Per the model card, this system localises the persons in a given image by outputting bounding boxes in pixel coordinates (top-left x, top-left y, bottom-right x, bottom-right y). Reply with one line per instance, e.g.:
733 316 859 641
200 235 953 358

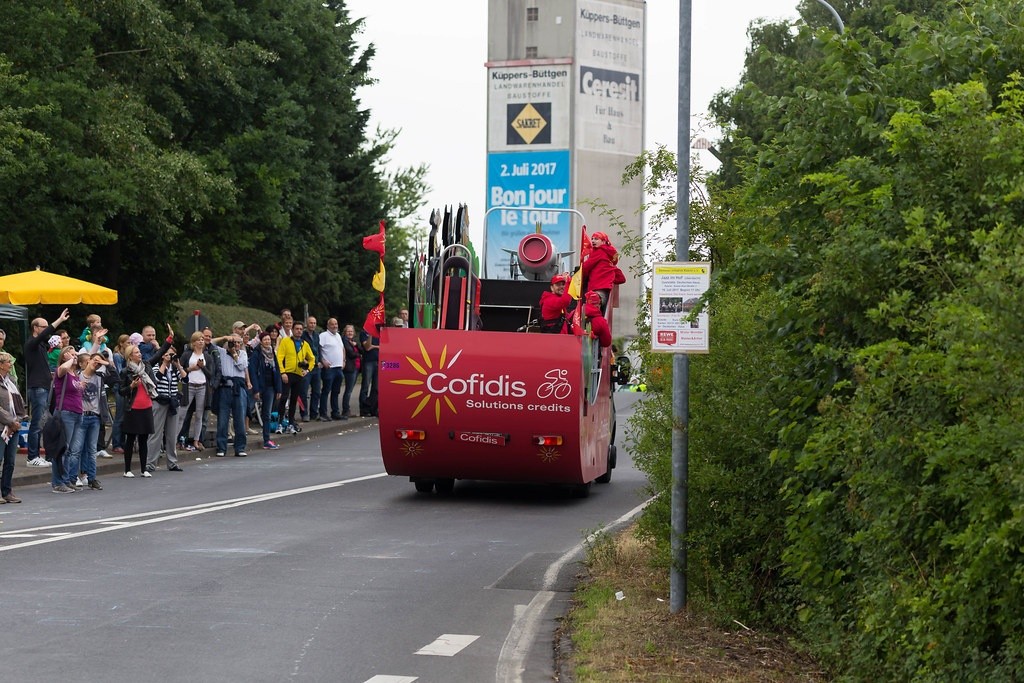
557 290 612 347
538 274 572 334
582 232 626 315
0 307 410 504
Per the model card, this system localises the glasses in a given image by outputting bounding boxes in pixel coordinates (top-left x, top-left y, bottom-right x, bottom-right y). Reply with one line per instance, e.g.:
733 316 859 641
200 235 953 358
38 326 47 329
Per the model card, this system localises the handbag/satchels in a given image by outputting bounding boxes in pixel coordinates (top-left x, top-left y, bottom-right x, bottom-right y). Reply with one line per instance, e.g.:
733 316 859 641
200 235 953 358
540 318 563 334
42 414 67 462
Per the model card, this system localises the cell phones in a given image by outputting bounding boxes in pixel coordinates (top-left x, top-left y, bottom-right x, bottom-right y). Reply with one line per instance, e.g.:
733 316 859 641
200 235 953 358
133 374 139 381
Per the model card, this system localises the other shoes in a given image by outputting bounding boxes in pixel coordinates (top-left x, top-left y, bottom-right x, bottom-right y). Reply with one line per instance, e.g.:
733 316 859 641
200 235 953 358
239 452 247 456
99 450 113 458
81 476 89 486
75 477 83 487
216 453 224 456
169 466 183 471
114 413 379 454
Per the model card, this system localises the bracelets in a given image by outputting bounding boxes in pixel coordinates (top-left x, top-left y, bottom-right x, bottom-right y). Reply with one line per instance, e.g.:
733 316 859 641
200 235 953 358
233 354 237 358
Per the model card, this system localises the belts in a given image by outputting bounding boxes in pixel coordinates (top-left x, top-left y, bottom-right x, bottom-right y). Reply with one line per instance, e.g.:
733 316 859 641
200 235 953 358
190 383 205 387
82 411 100 417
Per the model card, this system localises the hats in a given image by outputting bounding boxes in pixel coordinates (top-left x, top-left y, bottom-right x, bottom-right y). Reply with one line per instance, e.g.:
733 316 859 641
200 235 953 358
551 275 566 284
232 321 247 331
585 290 601 305
260 331 269 341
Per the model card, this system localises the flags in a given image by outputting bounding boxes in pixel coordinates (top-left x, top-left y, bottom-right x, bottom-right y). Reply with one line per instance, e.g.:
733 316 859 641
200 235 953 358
361 221 385 338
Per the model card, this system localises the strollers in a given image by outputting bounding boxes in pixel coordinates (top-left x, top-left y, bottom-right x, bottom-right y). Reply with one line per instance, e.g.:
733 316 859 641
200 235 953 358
250 395 302 433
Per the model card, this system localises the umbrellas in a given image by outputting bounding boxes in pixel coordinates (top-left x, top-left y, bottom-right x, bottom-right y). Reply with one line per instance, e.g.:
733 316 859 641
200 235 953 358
0 265 118 318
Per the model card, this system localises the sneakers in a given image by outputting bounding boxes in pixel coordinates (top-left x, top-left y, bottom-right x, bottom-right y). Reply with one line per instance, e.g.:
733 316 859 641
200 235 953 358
38 457 52 466
89 480 103 490
0 498 6 504
141 471 152 477
66 483 83 492
26 457 49 467
52 485 76 493
123 471 134 477
3 494 22 503
263 439 279 451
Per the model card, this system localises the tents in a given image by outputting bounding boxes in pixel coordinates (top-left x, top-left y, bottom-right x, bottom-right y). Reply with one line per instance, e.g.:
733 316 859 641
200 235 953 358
0 304 28 418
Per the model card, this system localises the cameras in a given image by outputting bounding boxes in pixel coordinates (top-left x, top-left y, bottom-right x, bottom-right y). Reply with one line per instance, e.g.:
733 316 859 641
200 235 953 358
228 341 234 349
169 353 181 363
298 362 309 370
